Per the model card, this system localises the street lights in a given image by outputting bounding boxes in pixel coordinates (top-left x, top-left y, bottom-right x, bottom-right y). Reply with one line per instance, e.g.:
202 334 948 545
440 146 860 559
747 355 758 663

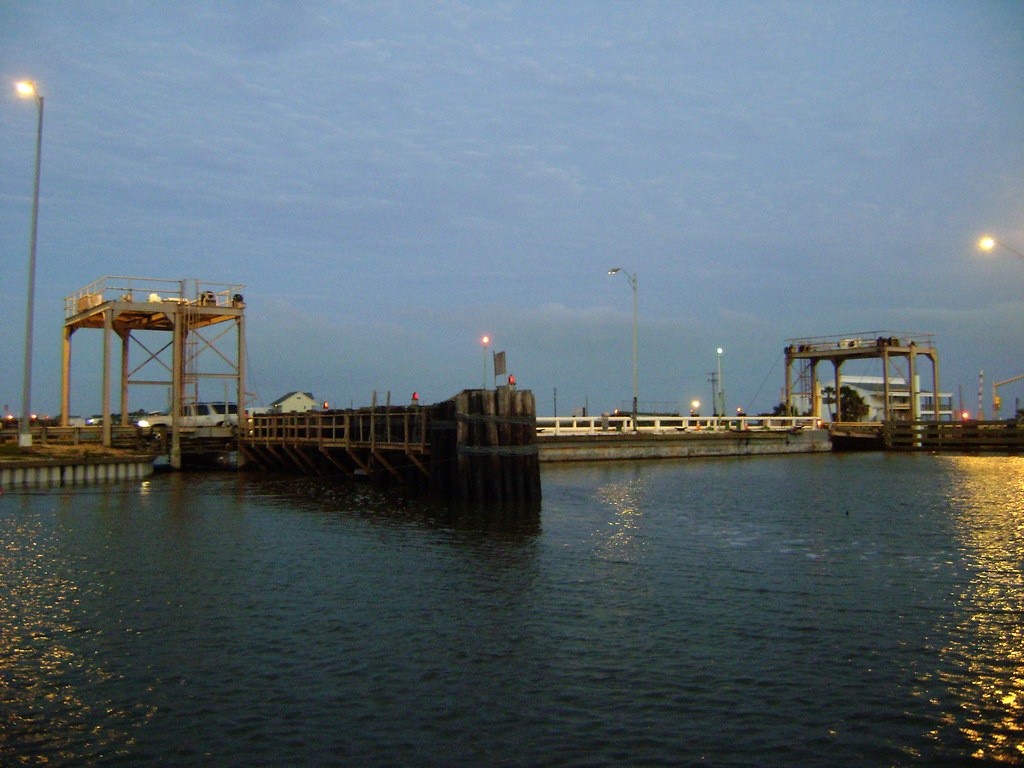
482 336 489 388
717 348 724 417
12 82 45 447
608 267 638 430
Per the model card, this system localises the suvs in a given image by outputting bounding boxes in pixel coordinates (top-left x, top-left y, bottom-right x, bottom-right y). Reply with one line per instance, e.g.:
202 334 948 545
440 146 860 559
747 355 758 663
135 400 247 437
